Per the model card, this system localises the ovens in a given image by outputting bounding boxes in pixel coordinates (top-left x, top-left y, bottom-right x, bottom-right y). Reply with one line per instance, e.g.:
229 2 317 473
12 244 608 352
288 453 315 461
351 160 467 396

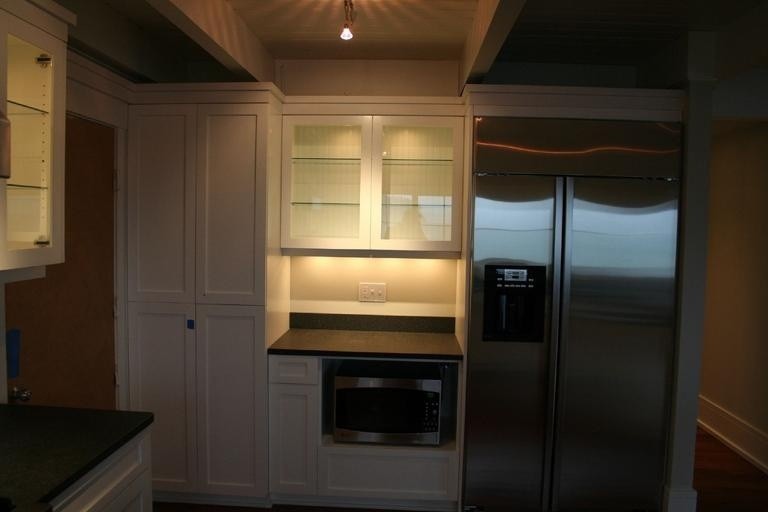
332 360 444 446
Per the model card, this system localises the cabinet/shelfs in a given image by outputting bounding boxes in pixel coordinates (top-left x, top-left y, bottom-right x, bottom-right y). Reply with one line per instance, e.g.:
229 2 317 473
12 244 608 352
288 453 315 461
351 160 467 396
122 83 293 509
0 0 79 273
268 351 464 512
280 96 466 254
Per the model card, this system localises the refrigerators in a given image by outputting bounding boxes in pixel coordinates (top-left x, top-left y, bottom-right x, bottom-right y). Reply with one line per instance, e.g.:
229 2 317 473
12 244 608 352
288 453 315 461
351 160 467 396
465 111 684 511
